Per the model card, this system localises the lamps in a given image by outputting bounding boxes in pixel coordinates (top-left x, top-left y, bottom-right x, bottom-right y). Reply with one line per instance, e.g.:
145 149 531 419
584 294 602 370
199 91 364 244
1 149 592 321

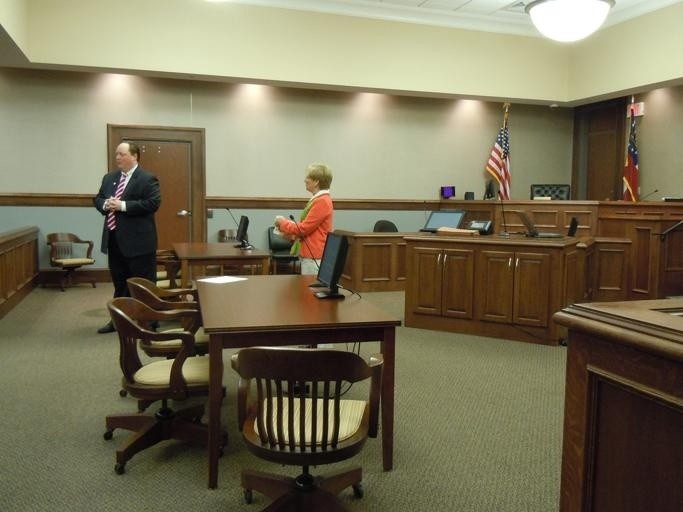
523 0 617 44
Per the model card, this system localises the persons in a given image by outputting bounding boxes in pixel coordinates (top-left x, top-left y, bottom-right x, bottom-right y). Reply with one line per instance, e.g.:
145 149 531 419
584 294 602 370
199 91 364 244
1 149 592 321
93 139 160 334
273 165 334 274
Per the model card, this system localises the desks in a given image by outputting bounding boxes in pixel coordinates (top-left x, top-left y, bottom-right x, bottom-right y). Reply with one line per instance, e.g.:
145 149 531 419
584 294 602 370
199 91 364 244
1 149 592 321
553 297 683 512
195 274 403 489
168 243 272 286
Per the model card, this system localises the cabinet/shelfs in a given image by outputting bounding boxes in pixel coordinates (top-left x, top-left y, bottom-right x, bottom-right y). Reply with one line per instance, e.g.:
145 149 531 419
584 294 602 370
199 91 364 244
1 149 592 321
334 229 409 295
403 235 577 346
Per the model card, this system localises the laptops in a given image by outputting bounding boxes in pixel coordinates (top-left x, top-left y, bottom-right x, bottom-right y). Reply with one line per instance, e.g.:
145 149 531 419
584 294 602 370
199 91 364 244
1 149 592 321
515 210 563 238
420 211 466 232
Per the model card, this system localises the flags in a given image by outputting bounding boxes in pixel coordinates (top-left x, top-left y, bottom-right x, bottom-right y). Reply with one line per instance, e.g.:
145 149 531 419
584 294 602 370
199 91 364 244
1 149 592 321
486 110 511 201
622 103 640 202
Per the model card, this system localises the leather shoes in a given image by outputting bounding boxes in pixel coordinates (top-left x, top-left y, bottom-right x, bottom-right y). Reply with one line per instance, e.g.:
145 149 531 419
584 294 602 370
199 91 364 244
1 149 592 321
98 318 116 334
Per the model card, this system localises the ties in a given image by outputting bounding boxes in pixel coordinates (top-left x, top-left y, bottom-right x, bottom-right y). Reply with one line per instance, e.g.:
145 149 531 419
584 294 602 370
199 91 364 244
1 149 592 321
106 173 128 232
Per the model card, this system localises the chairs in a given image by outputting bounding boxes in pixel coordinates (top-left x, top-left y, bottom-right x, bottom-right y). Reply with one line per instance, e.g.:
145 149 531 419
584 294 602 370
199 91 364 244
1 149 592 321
38 232 96 292
119 278 226 403
105 297 228 476
568 217 578 236
266 228 301 273
224 349 393 511
529 184 570 200
151 249 182 290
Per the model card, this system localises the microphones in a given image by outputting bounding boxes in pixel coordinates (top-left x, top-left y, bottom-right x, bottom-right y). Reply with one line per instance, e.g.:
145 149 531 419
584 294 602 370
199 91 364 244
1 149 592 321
226 207 254 250
497 190 508 236
637 189 658 202
288 215 327 288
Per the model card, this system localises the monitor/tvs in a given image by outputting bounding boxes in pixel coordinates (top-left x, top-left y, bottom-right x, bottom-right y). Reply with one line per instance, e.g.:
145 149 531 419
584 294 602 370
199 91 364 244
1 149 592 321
233 216 249 248
314 232 350 299
